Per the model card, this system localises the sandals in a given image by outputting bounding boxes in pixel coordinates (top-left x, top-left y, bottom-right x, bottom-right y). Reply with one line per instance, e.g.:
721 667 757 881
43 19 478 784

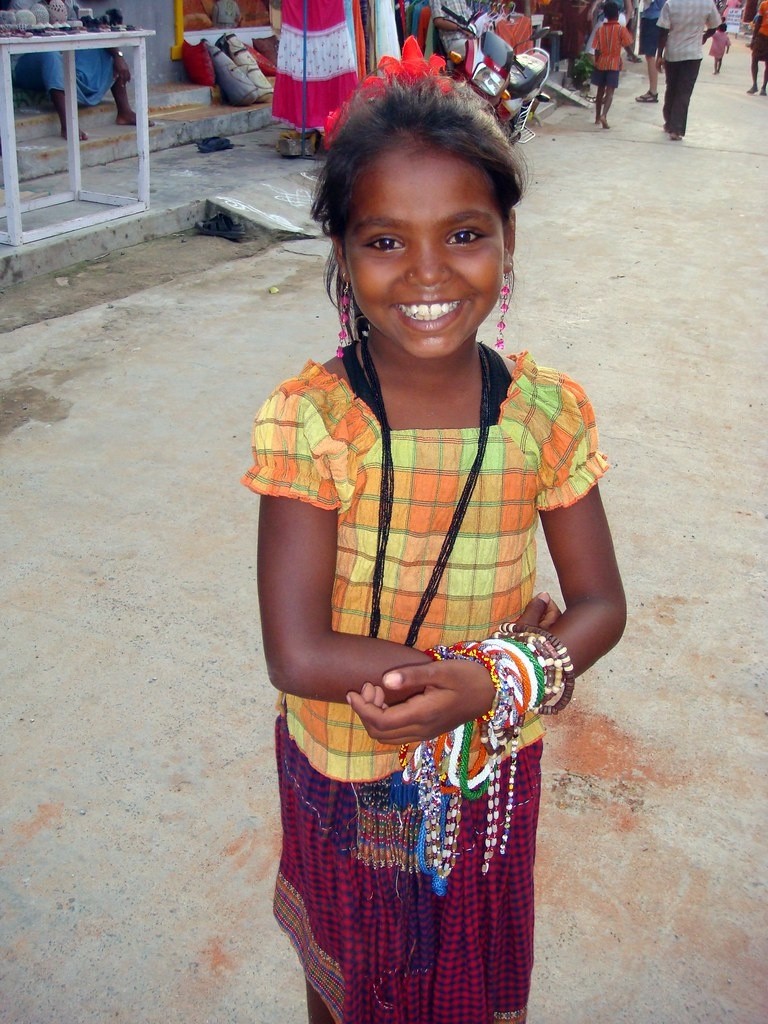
635 90 659 102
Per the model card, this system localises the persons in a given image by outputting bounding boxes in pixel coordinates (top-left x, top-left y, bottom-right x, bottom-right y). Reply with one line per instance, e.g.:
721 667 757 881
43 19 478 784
591 3 638 129
709 23 731 74
8 0 155 141
747 0 768 96
635 0 667 103
242 35 628 1024
655 0 722 140
608 0 641 62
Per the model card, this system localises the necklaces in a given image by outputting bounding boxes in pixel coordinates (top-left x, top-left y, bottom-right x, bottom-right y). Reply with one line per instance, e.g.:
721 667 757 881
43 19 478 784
398 623 575 897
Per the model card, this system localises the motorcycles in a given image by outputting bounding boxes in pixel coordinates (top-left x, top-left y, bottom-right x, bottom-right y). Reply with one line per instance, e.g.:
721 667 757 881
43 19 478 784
439 5 550 147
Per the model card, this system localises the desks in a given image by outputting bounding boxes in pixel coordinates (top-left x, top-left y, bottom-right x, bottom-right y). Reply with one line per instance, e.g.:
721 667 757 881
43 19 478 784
0 29 156 244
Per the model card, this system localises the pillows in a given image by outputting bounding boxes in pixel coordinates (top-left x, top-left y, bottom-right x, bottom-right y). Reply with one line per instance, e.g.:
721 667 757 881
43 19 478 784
243 42 276 77
208 33 273 107
251 35 277 66
182 39 215 87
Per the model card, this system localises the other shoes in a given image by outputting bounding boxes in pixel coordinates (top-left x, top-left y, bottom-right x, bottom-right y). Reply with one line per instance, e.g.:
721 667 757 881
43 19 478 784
627 56 642 63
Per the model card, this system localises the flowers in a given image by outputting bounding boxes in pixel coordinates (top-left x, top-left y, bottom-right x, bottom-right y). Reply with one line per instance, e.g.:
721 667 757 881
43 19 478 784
324 35 451 151
